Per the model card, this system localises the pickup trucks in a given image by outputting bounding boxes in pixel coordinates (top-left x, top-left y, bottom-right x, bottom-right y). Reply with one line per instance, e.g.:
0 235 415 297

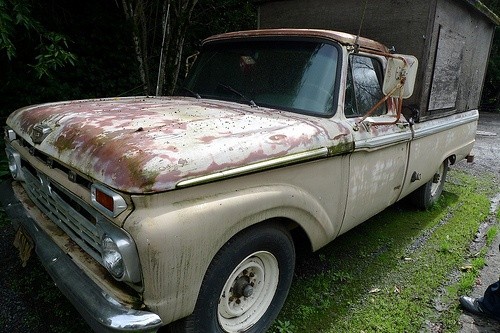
9 27 479 333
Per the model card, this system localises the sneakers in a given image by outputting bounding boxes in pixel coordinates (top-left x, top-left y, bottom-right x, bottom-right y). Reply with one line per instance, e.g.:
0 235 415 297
459 296 488 317
